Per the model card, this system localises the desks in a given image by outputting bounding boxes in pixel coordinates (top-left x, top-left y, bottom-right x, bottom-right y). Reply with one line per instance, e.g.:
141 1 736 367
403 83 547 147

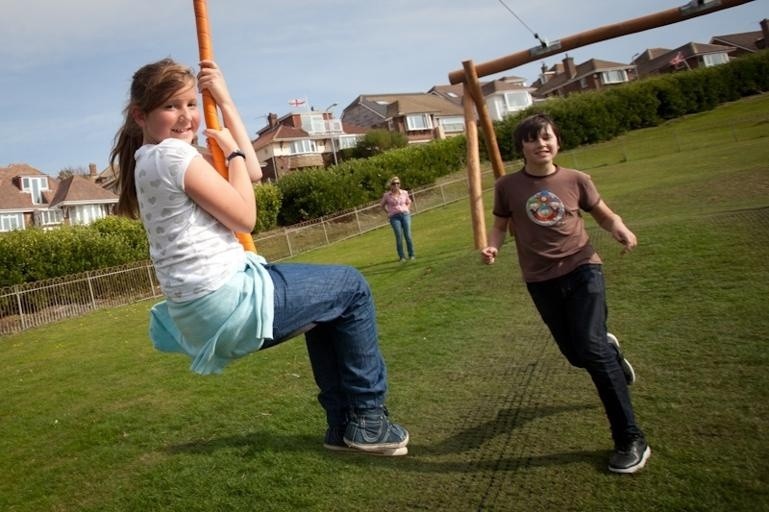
325 102 339 167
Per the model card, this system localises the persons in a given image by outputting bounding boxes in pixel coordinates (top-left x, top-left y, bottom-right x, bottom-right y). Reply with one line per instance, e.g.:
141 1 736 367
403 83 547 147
108 57 412 456
381 176 414 263
481 111 652 473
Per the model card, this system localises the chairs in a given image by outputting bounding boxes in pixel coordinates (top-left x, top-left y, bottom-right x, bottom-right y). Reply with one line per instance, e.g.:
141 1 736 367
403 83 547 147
601 331 635 386
607 435 652 474
323 420 411 456
338 406 411 449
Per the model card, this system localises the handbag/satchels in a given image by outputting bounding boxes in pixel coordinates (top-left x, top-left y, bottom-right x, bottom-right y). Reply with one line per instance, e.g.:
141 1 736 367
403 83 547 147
224 148 247 166
